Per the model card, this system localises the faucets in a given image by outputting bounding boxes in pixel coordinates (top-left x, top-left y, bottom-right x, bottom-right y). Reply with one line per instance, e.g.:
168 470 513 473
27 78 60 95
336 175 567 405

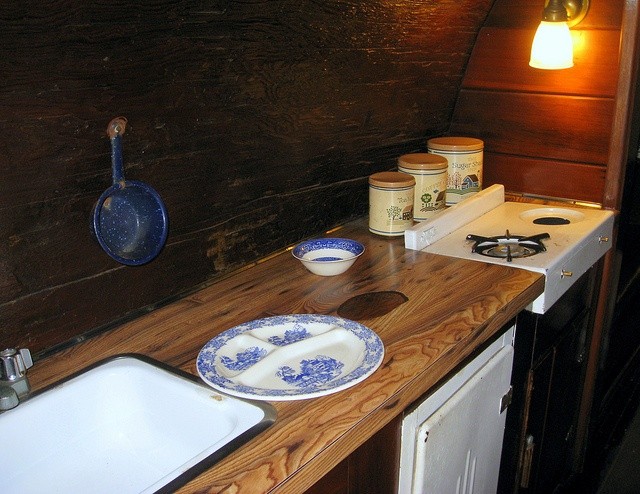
0 346 33 411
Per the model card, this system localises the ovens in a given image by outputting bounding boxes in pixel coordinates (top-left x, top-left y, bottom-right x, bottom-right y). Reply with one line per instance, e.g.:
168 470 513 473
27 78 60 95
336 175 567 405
497 254 606 494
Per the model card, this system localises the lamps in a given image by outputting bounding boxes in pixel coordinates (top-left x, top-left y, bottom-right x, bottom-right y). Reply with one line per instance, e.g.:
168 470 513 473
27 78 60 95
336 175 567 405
527 0 591 74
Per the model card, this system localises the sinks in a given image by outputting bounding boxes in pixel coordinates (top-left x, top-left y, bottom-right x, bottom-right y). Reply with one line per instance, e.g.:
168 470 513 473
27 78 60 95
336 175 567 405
0 357 265 494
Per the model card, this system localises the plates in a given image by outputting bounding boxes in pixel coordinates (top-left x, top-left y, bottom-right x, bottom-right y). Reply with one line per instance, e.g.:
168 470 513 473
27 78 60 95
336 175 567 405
196 312 385 401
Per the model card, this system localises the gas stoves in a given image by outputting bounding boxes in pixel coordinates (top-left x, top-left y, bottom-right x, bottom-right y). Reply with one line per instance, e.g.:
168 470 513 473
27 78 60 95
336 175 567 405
421 201 616 315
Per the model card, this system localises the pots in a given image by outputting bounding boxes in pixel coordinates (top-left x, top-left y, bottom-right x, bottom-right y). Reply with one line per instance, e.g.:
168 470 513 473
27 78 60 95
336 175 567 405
87 117 170 267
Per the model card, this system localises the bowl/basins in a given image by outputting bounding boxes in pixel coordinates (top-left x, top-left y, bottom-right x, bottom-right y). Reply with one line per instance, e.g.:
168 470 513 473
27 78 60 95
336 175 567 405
291 238 366 277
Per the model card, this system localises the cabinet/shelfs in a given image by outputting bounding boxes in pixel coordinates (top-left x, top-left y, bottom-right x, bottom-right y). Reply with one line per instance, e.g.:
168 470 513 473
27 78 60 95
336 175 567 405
303 318 544 492
513 257 607 492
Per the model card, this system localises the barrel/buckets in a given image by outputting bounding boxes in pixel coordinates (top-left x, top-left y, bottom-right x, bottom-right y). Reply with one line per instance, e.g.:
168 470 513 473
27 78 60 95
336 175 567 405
427 136 486 206
397 152 447 221
367 169 415 238
